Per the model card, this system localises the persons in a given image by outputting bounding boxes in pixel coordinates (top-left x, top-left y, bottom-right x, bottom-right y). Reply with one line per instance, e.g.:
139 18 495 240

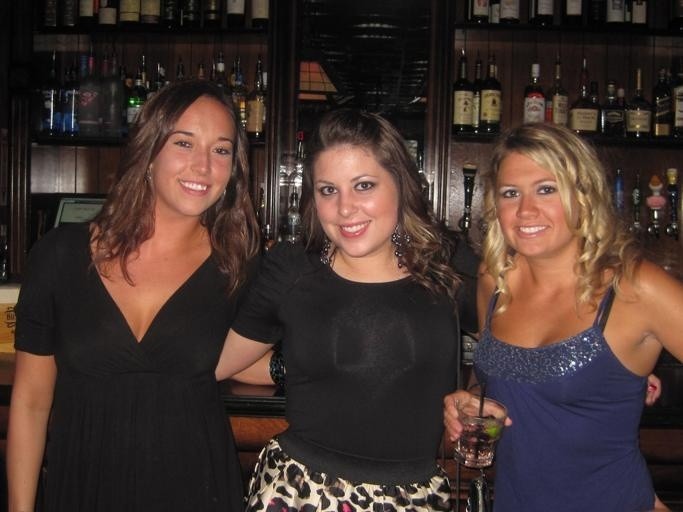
213 97 664 512
442 122 682 511
3 79 290 509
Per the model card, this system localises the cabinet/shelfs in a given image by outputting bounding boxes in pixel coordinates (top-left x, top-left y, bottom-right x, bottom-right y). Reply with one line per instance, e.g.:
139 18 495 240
0 1 683 335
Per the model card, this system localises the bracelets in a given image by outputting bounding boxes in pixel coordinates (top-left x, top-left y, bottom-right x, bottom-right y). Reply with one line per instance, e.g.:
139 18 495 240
267 351 285 390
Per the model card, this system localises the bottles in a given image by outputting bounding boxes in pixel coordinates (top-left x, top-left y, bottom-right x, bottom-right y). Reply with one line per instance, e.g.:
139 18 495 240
39 0 270 143
452 46 683 139
282 126 314 247
464 0 652 32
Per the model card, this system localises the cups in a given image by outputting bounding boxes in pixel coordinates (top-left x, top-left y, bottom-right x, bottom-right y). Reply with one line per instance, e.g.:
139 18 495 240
452 394 508 470
